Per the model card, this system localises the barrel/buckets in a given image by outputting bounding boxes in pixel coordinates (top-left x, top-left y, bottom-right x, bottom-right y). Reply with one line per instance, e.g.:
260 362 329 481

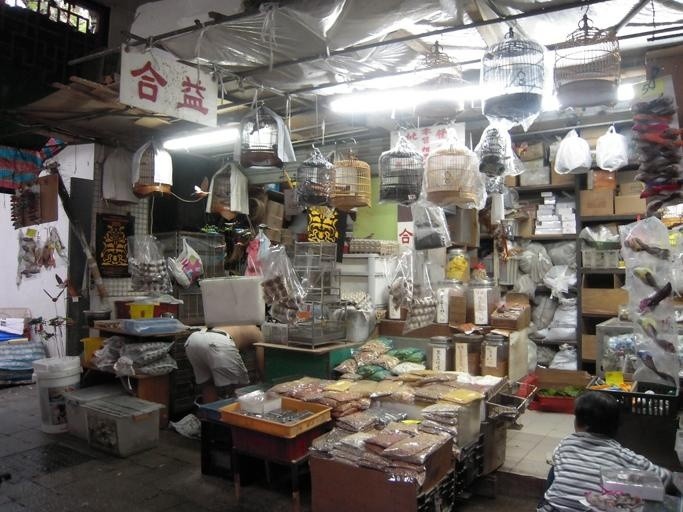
32 356 82 434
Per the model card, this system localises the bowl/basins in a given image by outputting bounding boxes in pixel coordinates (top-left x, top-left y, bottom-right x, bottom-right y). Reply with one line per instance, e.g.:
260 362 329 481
83 309 111 328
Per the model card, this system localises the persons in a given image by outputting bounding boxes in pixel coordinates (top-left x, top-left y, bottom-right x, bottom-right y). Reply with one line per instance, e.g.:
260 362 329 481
183 324 264 404
534 389 672 512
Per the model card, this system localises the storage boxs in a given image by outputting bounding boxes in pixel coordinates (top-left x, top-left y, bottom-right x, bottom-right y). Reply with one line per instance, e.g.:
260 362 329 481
486 125 650 390
63 380 122 442
532 387 577 411
483 415 510 476
414 435 462 496
84 395 165 457
151 185 448 417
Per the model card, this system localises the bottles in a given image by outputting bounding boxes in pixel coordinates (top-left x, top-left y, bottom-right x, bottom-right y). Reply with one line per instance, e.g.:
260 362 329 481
436 281 500 326
429 333 508 378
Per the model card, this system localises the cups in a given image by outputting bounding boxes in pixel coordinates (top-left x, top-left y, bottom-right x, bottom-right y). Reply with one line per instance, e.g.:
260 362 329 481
80 337 101 364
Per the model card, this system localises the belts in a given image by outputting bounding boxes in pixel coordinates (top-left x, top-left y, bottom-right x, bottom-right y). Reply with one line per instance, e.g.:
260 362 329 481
190 328 233 340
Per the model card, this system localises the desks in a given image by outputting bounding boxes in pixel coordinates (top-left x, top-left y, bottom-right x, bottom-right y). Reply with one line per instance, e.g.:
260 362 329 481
224 429 334 510
80 366 169 427
